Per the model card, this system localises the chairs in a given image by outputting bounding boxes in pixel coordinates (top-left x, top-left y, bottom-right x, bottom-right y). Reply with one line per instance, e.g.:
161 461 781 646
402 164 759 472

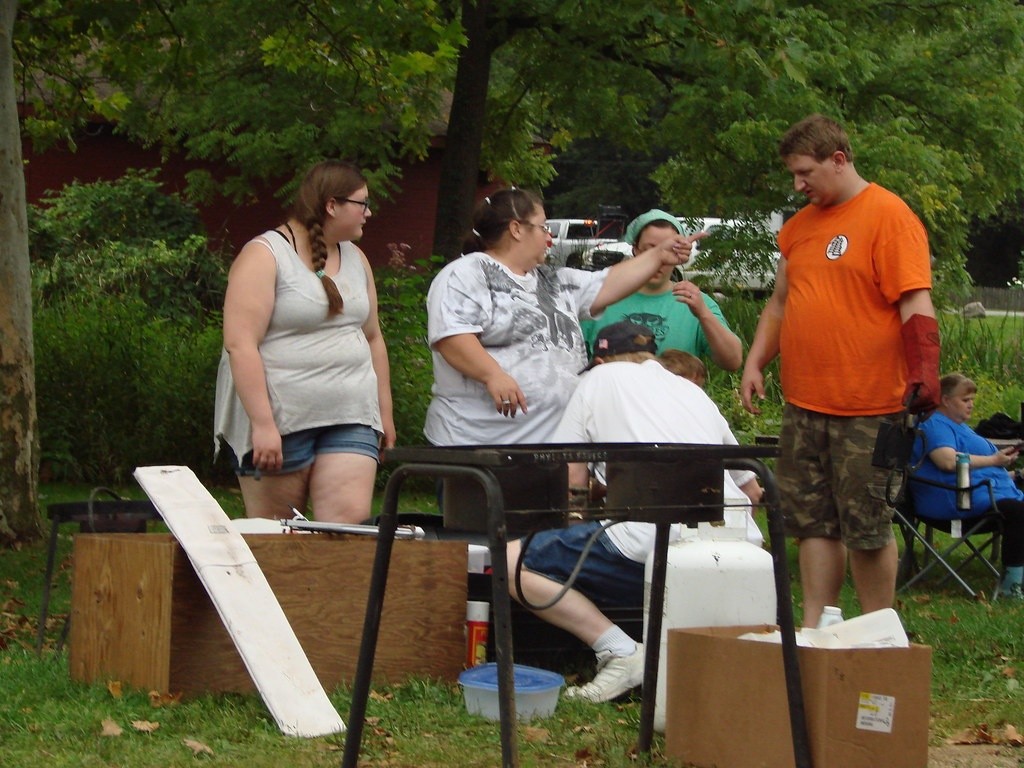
888 470 1002 599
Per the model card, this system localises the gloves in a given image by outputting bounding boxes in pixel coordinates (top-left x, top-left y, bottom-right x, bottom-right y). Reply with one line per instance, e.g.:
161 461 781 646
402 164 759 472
901 314 941 422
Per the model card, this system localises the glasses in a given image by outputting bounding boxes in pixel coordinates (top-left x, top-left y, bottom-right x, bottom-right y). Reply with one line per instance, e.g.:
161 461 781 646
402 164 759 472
518 219 551 234
339 198 371 214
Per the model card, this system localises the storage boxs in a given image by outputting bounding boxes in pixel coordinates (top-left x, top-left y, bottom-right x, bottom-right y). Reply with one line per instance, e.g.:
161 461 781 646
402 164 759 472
69 531 469 704
664 622 932 768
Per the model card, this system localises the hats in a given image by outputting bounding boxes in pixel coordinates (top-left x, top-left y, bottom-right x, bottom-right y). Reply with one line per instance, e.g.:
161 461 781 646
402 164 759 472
576 322 658 375
625 209 687 244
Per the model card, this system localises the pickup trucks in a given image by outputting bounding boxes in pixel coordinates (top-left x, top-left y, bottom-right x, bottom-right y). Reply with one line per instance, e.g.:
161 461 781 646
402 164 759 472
581 217 781 301
544 217 619 270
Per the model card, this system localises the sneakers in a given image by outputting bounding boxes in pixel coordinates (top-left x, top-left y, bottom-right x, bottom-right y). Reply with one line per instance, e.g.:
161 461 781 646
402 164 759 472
563 643 644 704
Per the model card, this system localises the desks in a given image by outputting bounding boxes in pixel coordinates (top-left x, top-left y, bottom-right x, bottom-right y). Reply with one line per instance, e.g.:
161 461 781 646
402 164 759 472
922 438 1024 566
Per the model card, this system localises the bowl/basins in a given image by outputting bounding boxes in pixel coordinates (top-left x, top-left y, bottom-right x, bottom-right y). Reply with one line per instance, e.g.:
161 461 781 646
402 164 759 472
457 664 566 719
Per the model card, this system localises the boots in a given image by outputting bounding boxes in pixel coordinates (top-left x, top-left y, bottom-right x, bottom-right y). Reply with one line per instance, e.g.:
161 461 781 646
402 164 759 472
1000 566 1024 599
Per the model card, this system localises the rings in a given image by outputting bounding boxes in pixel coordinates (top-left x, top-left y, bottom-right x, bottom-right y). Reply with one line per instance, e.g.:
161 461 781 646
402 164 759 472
504 400 511 404
690 294 692 298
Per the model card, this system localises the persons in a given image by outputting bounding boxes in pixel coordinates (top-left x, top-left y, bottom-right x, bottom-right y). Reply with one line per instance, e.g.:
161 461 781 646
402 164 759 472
506 322 766 704
906 371 1024 596
579 209 743 372
423 187 709 516
211 162 398 524
740 112 942 629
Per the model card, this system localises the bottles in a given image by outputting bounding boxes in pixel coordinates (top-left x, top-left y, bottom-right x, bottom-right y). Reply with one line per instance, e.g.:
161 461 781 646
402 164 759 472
956 454 970 509
